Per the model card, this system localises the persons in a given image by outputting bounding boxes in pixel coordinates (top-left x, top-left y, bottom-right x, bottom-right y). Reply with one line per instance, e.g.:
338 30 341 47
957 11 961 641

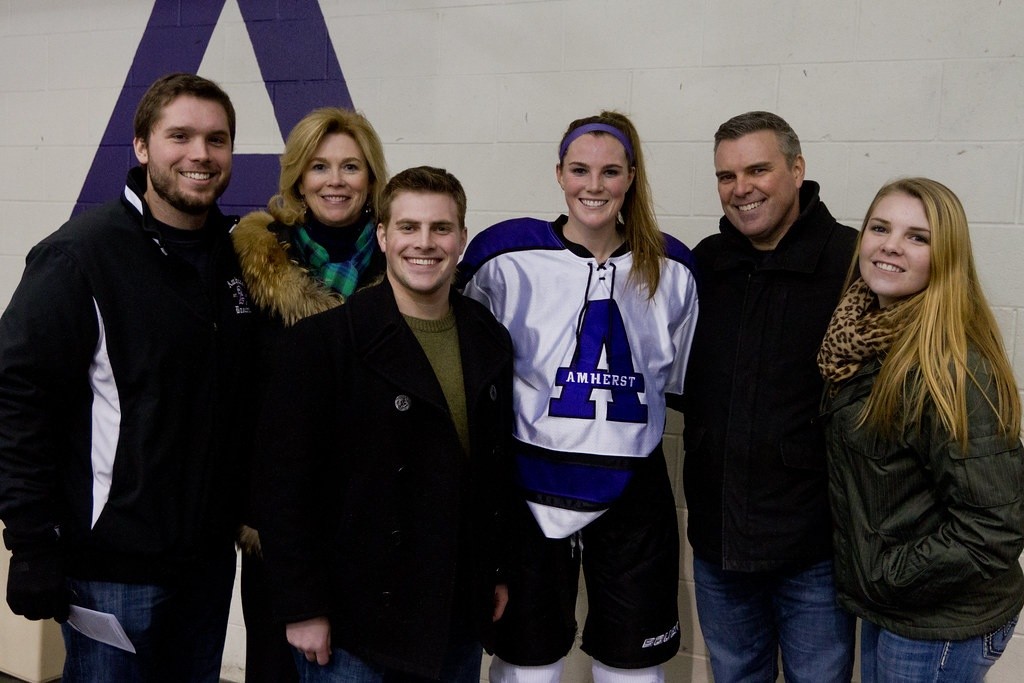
0 72 239 683
455 110 701 682
811 174 1024 682
224 106 512 683
685 110 861 666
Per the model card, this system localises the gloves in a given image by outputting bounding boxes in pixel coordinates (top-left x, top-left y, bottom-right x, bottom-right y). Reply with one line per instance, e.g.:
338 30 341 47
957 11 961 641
1 526 81 625
